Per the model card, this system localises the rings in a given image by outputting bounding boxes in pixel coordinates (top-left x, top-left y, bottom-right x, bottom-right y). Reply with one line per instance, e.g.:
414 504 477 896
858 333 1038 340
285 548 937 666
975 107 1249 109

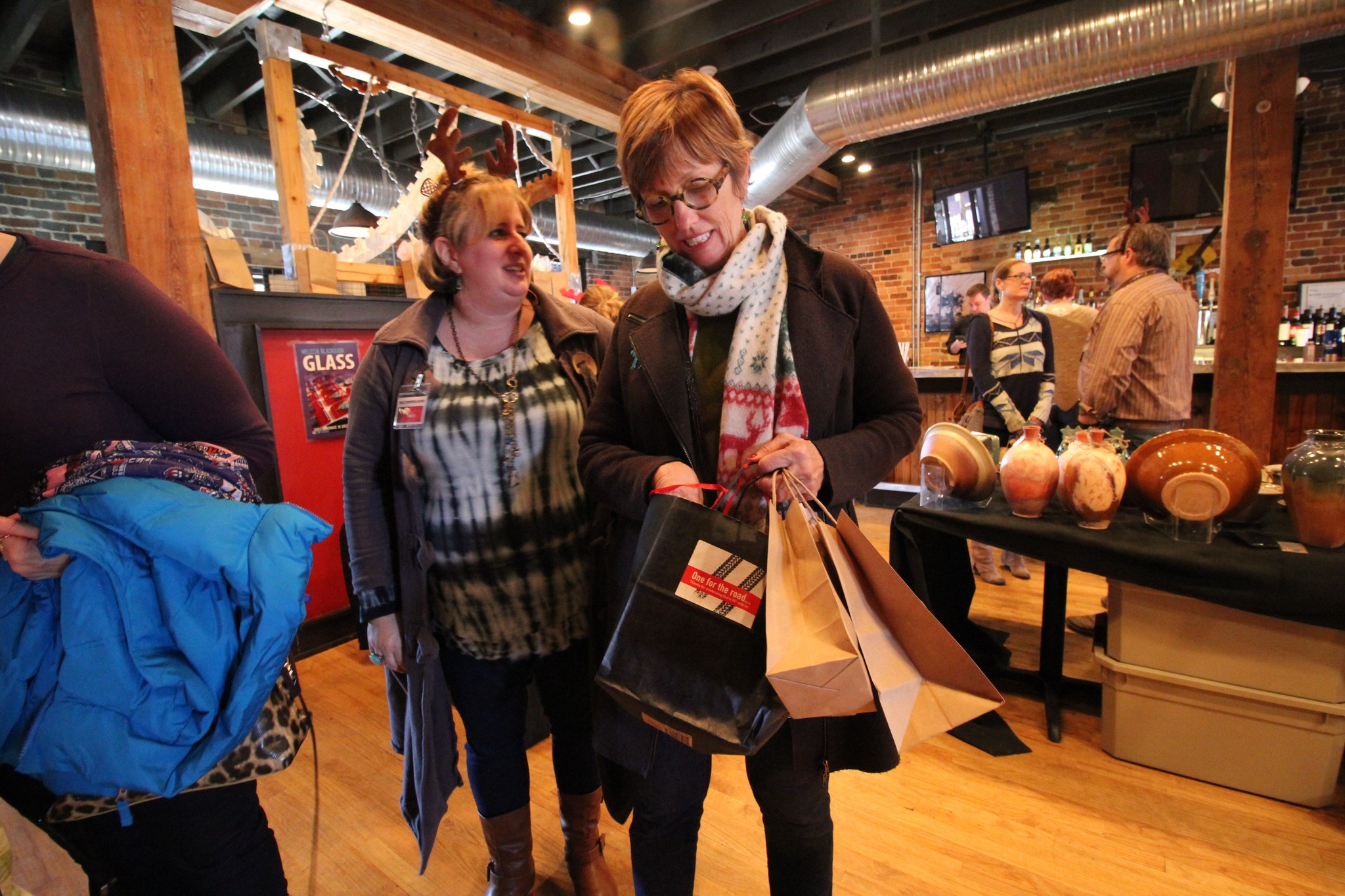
368 653 385 665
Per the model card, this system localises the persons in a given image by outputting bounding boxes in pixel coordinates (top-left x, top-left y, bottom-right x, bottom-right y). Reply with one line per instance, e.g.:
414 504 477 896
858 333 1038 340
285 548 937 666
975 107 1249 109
568 67 922 894
944 284 995 366
580 284 623 325
339 162 622 896
1030 268 1098 430
1067 222 1199 640
0 226 289 896
966 257 1060 584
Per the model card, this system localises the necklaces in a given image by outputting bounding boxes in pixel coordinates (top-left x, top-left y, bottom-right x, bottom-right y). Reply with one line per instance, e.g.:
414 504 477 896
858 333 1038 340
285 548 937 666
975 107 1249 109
446 297 525 489
989 312 1022 372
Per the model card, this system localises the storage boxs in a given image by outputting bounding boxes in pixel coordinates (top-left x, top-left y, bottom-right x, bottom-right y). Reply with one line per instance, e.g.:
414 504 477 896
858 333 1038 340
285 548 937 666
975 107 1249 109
1100 577 1345 705
1091 640 1345 810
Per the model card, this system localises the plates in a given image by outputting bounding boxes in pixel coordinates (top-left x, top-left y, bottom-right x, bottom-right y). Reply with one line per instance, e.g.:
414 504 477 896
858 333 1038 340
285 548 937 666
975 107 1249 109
1258 467 1284 494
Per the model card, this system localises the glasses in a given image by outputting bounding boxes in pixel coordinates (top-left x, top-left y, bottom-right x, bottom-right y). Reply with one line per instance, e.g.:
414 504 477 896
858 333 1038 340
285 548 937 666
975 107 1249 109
1002 272 1033 280
1099 248 1125 261
635 161 731 226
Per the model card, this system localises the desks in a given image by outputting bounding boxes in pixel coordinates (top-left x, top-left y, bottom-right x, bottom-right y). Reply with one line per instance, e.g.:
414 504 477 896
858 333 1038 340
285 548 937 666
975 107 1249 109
895 481 1345 745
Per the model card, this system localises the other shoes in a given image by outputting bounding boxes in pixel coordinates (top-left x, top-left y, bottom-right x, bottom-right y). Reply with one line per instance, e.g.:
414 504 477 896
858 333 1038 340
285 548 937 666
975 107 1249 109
1064 610 1108 635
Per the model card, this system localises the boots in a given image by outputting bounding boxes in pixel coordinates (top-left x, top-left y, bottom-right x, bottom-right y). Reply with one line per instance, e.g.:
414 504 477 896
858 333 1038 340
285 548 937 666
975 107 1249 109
476 797 536 896
970 539 1006 586
555 783 620 896
1001 549 1030 580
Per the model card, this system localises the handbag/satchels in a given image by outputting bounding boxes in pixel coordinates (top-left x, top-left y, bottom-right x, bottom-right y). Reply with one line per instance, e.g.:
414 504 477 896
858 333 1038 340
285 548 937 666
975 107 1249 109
41 645 313 824
951 399 984 434
783 467 1005 756
595 460 789 755
767 470 878 721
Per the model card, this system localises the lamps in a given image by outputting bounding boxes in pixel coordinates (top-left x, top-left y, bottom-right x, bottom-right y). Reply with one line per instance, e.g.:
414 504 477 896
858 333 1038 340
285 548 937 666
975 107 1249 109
1295 77 1311 99
1210 92 1230 113
567 0 598 27
327 76 381 241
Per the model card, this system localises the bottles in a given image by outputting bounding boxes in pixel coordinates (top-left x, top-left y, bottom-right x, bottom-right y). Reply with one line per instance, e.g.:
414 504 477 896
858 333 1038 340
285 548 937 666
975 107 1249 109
1278 302 1345 358
1023 287 1044 308
1014 233 1092 260
1282 429 1345 548
1000 425 1127 530
1077 286 1105 310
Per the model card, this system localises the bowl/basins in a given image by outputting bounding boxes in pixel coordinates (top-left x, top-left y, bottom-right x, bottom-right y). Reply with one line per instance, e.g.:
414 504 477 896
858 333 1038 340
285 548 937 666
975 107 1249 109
918 422 997 499
1125 429 1261 523
